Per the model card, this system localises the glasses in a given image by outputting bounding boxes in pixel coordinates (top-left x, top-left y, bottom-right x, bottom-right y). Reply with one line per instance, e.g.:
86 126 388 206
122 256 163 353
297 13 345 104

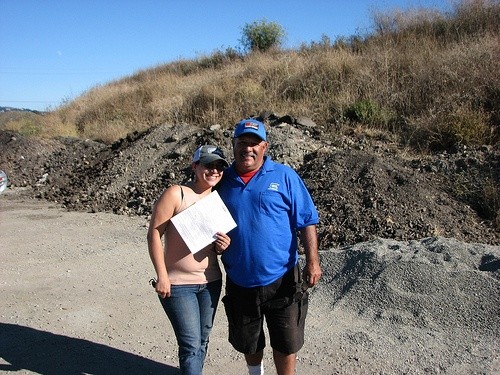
201 163 225 172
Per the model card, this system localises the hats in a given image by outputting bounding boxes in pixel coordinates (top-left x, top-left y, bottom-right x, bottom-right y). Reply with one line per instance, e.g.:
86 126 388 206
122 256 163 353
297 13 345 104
193 145 230 168
234 119 267 143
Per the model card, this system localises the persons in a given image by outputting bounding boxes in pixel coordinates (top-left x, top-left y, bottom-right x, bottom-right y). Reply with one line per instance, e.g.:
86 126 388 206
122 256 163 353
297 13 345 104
212 118 322 375
147 144 232 375
